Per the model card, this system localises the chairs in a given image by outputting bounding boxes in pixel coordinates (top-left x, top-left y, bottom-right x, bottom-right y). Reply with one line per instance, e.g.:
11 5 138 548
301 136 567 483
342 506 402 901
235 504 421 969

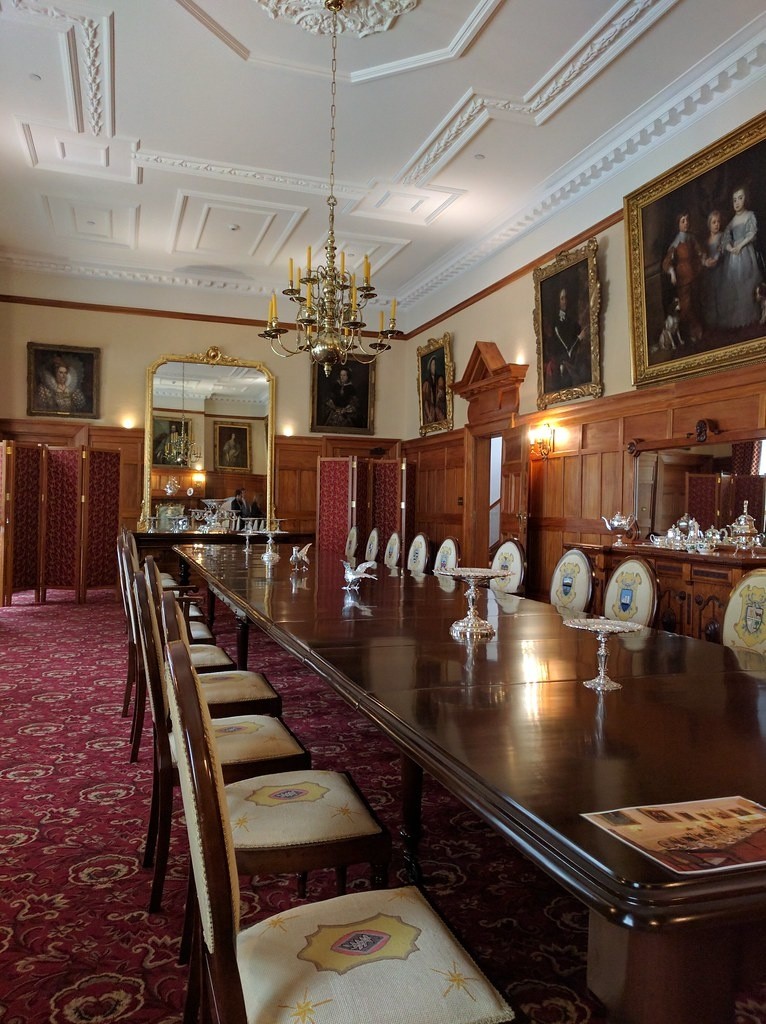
111 530 766 1023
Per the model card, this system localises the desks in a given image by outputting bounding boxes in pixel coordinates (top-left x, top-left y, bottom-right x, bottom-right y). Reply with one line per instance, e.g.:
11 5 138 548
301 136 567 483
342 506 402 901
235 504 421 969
133 532 316 561
173 543 766 1024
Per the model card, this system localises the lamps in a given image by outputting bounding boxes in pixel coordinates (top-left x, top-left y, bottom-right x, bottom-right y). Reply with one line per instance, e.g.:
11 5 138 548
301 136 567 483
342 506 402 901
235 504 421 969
528 424 552 461
256 1 405 378
163 361 202 468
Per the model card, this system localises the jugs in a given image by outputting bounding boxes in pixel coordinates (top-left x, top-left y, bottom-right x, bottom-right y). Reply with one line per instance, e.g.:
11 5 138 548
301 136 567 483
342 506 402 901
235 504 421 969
602 511 636 546
700 525 728 543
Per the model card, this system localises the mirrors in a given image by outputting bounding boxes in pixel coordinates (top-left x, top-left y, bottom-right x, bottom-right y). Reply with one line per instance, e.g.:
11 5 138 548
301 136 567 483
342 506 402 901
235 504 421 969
136 346 279 533
628 418 766 555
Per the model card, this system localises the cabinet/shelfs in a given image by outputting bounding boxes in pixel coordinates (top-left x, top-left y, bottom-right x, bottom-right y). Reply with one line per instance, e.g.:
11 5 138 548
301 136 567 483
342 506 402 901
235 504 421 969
563 541 766 646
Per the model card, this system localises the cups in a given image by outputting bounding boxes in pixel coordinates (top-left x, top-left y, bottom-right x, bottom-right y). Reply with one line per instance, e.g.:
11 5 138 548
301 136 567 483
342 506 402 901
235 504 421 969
697 543 716 555
650 534 666 546
686 543 697 554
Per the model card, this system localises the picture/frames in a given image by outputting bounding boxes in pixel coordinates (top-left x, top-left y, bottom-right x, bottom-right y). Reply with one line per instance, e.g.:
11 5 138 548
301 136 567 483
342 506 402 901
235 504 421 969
416 332 455 436
309 353 376 435
152 415 192 467
213 421 253 474
532 237 603 410
622 109 766 388
26 341 100 419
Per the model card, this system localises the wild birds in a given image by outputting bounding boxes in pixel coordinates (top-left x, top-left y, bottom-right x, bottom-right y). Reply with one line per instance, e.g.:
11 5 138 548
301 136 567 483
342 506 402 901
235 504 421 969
290 543 313 572
339 560 378 590
